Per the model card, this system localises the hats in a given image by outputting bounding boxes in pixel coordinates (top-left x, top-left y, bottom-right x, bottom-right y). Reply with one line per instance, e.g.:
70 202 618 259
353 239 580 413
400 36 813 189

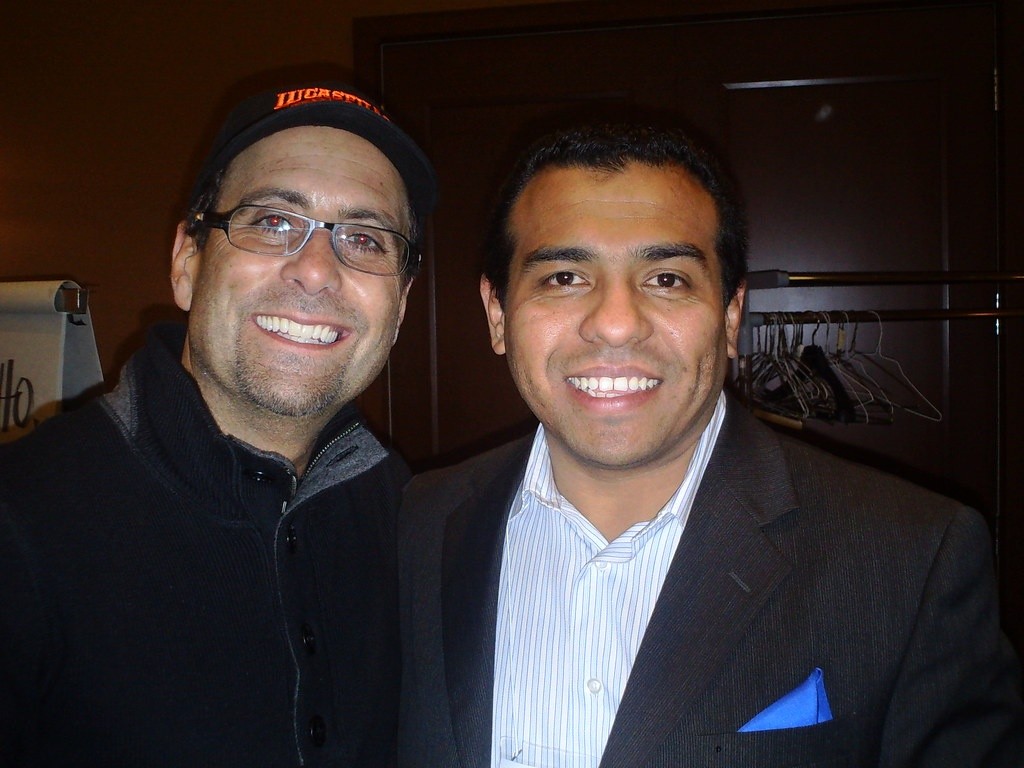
188 84 435 215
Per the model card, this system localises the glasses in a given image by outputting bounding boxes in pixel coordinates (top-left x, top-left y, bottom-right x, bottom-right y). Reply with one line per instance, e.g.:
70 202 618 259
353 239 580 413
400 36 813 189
193 203 421 277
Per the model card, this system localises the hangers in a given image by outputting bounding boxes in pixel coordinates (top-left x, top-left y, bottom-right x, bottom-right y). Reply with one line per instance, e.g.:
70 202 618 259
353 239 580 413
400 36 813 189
725 311 943 430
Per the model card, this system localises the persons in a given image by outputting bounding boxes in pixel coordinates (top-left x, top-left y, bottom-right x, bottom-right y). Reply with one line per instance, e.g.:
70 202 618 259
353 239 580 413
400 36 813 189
393 120 1024 768
0 59 433 768
